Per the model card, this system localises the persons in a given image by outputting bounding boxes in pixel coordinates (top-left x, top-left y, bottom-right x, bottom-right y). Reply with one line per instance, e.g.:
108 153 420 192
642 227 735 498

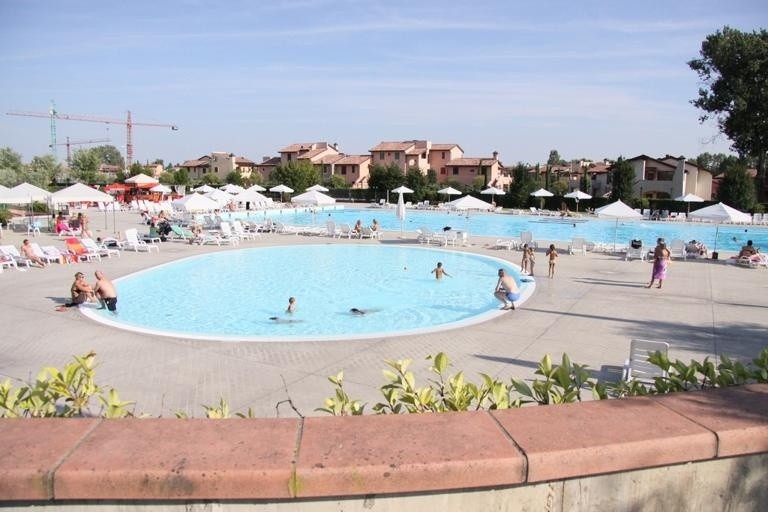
521 244 535 276
494 269 520 310
740 240 756 253
649 238 670 288
77 212 85 231
546 244 558 278
70 271 117 311
21 239 47 269
355 220 361 232
50 211 70 232
288 297 299 314
372 219 378 231
431 262 451 280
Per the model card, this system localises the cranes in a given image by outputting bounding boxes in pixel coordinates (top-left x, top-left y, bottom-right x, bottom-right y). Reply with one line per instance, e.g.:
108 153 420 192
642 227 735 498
5 98 179 175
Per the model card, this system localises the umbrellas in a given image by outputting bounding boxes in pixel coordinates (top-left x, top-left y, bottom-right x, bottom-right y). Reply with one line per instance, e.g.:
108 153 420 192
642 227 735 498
103 173 336 239
0 180 114 225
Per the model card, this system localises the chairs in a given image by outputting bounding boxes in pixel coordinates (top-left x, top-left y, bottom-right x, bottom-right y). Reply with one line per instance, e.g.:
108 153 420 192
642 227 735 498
492 230 537 252
669 238 688 260
264 214 386 240
624 239 645 261
621 338 670 396
116 228 160 254
1 214 122 269
145 214 264 247
415 227 471 249
567 236 588 257
